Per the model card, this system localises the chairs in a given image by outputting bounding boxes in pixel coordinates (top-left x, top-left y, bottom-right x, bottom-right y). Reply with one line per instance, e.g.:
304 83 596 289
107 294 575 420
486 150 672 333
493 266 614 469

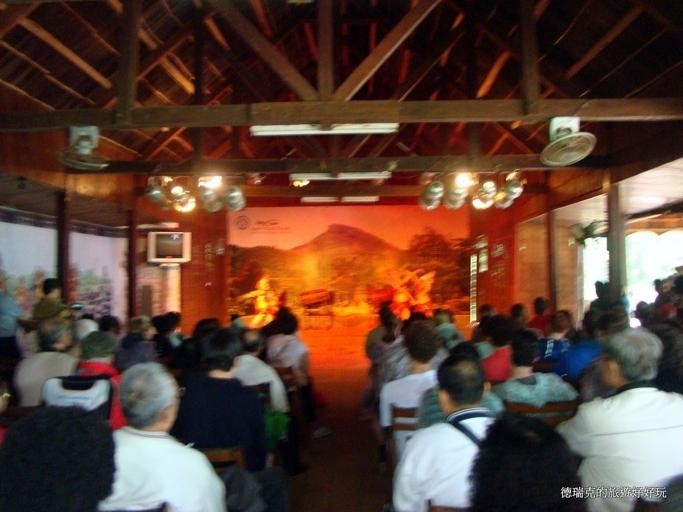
506 400 579 431
204 446 245 479
276 364 303 413
386 406 419 485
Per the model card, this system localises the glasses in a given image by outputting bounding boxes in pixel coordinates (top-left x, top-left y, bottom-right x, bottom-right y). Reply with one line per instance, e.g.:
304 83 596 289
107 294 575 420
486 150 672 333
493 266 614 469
175 387 186 397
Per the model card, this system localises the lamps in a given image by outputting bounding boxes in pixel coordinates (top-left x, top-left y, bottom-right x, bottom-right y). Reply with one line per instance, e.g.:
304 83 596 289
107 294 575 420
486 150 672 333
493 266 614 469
249 122 399 135
471 180 497 210
420 181 444 210
445 171 480 208
224 186 246 211
495 169 524 210
289 170 392 180
198 176 224 212
146 176 196 212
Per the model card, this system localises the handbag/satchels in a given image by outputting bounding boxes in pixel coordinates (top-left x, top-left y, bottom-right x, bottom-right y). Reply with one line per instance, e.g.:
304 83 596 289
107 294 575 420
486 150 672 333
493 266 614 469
447 420 482 447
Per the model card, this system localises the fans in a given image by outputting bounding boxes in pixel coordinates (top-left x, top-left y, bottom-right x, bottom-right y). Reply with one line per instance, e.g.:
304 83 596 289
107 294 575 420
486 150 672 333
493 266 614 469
58 126 109 169
540 116 596 167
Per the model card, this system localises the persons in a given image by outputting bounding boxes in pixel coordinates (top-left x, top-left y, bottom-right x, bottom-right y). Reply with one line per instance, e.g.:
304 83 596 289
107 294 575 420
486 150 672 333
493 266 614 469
261 308 288 336
14 317 79 407
499 333 579 415
0 403 115 511
537 310 573 361
473 323 495 358
98 360 228 511
68 318 99 356
71 331 126 429
416 340 506 425
526 296 553 336
169 332 290 510
194 319 220 336
118 316 161 370
365 303 464 382
264 317 318 422
466 409 584 511
231 329 306 475
556 320 599 381
471 304 495 340
30 279 70 322
82 315 121 358
582 266 683 387
0 372 13 445
553 329 682 512
391 352 497 512
159 312 186 365
478 316 513 383
508 303 526 324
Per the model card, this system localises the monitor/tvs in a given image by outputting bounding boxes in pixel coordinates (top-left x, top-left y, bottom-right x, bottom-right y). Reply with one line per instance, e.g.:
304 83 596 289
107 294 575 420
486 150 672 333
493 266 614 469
146 231 191 266
